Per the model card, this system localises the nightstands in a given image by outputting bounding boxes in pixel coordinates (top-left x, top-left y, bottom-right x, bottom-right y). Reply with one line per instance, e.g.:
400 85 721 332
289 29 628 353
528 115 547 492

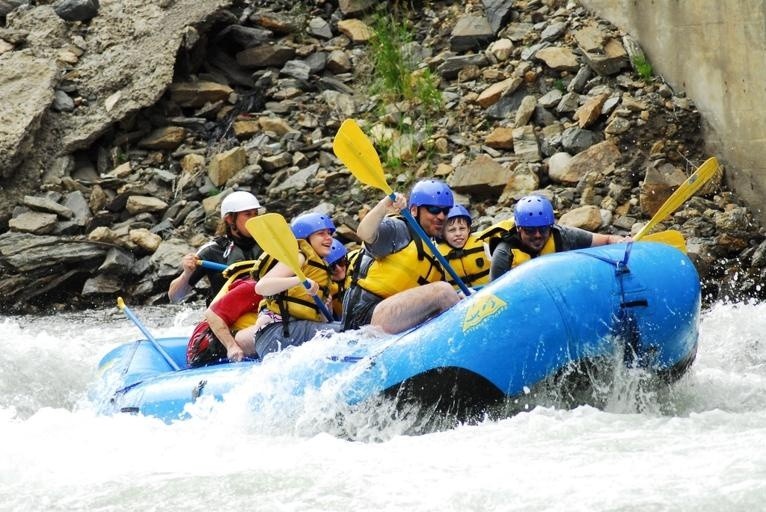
333 118 471 298
246 213 335 320
633 158 719 240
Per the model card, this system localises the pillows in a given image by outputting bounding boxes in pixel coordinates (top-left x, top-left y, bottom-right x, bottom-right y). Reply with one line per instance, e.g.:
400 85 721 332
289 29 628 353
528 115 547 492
220 191 261 218
514 195 555 226
408 177 453 210
448 205 472 223
323 238 348 266
291 212 336 240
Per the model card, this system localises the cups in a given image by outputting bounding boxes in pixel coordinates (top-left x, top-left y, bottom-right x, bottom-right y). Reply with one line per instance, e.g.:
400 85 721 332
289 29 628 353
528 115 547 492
331 258 345 267
524 227 549 235
425 206 449 215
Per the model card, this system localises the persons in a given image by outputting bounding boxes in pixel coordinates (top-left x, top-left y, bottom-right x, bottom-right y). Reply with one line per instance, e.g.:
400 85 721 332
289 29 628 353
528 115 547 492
490 195 633 283
186 275 263 364
322 238 361 321
254 212 341 360
169 191 263 309
434 203 492 291
340 179 478 336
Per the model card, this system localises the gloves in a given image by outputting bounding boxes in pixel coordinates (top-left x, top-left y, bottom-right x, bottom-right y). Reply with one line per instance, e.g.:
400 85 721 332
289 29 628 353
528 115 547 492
90 238 702 437
